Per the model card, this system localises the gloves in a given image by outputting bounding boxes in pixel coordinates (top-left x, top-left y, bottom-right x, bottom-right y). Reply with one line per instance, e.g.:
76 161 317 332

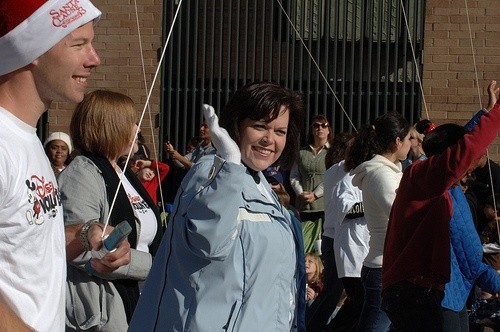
200 103 241 165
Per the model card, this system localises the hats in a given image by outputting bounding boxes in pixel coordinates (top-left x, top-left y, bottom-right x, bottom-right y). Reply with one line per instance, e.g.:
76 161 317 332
43 131 73 156
0 0 103 80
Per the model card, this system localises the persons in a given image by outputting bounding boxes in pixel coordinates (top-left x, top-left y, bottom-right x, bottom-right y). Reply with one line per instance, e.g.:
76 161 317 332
43 131 72 177
127 82 307 332
118 125 294 236
302 252 327 309
0 0 101 332
55 89 163 331
349 111 412 331
331 135 425 331
304 134 357 332
382 80 500 332
165 118 216 168
290 114 342 255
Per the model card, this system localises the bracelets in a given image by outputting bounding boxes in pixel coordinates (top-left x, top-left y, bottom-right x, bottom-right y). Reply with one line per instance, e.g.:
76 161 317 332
79 219 96 253
313 192 319 200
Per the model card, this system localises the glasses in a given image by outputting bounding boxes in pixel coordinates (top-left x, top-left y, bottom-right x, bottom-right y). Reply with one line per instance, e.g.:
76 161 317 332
311 122 329 129
